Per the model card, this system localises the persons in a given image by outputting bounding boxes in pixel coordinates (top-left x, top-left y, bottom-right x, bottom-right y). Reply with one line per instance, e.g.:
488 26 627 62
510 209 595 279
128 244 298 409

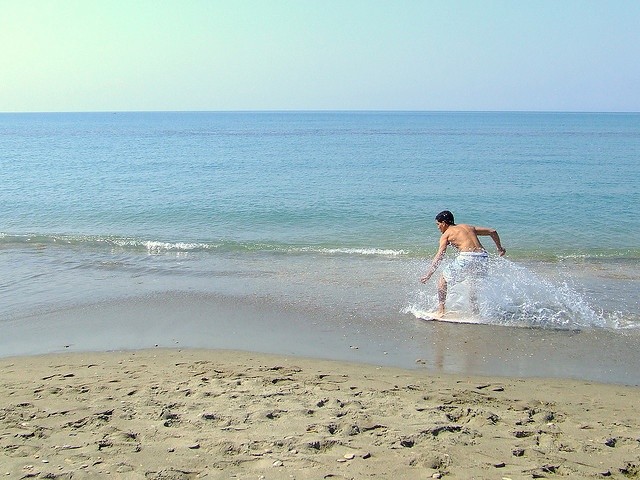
421 210 506 316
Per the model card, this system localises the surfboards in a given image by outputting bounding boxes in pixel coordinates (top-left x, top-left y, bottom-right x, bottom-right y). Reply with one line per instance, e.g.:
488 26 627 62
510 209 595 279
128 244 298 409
412 309 583 331
